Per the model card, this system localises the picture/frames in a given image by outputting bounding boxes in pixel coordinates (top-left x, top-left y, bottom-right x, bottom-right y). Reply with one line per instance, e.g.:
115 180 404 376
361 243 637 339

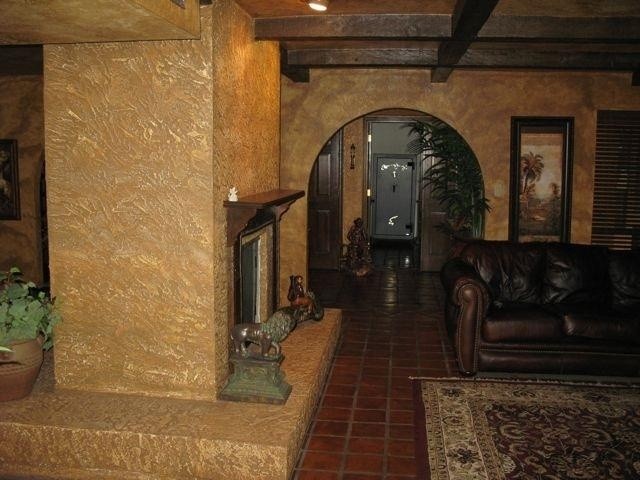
0 139 22 221
507 115 575 244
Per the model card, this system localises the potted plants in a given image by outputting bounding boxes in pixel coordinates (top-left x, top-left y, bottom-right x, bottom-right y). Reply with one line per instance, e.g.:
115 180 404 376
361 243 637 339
0 262 64 403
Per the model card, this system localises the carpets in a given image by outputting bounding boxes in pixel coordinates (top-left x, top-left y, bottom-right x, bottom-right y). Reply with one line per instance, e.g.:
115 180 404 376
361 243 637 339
407 374 640 480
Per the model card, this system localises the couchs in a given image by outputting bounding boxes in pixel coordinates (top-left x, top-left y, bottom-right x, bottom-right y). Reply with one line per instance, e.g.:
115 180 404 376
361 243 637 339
439 237 640 379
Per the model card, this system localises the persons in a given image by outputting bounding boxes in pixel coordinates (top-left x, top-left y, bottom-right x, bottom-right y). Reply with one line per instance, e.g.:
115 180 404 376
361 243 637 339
347 218 368 259
290 274 313 314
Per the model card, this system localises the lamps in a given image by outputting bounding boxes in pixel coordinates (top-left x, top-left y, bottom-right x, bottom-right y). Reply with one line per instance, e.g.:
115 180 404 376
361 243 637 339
350 144 356 169
308 0 330 12
